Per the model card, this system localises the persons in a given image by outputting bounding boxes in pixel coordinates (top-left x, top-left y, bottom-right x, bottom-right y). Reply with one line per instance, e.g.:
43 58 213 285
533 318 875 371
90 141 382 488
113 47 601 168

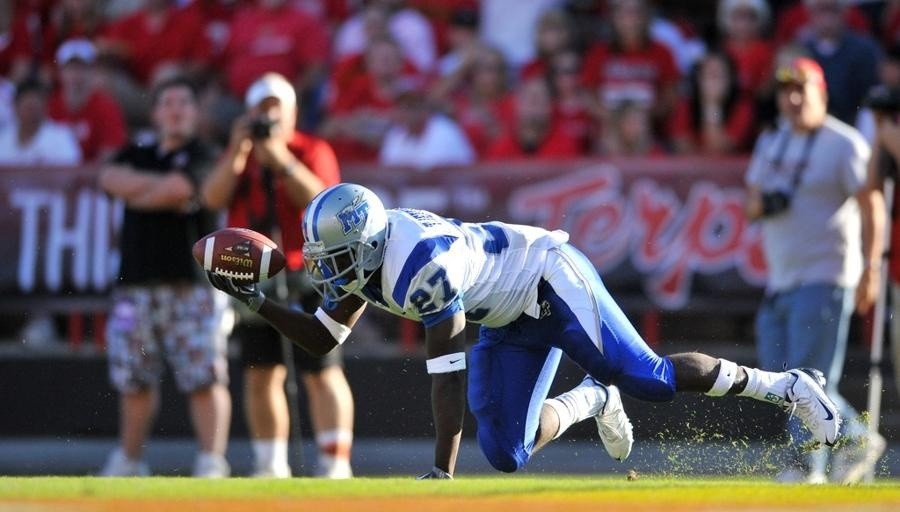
0 0 900 353
873 89 900 400
209 180 844 481
740 55 889 485
202 69 362 480
90 71 238 480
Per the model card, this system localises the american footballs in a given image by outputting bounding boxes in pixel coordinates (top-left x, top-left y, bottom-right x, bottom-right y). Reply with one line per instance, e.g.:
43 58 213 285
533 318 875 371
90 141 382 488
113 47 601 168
192 228 287 286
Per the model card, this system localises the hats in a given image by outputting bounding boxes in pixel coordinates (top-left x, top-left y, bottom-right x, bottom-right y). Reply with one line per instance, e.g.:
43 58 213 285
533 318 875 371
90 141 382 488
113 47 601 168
240 69 297 110
769 52 826 89
53 35 98 71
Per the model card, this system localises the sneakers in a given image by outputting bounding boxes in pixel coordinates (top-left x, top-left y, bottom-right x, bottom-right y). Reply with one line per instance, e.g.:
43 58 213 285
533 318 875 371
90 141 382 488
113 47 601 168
580 372 640 465
776 360 843 449
830 424 887 487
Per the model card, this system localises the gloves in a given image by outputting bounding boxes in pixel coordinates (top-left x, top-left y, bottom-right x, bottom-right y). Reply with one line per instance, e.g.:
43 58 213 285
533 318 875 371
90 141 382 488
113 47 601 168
201 267 268 316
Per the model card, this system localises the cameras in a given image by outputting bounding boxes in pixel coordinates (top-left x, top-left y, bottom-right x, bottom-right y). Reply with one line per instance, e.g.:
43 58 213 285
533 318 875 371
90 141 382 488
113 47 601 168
763 190 788 216
245 116 277 141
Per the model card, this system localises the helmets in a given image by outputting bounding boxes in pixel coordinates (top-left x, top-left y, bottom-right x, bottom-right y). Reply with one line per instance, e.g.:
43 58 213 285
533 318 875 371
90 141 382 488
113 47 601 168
296 179 390 310
